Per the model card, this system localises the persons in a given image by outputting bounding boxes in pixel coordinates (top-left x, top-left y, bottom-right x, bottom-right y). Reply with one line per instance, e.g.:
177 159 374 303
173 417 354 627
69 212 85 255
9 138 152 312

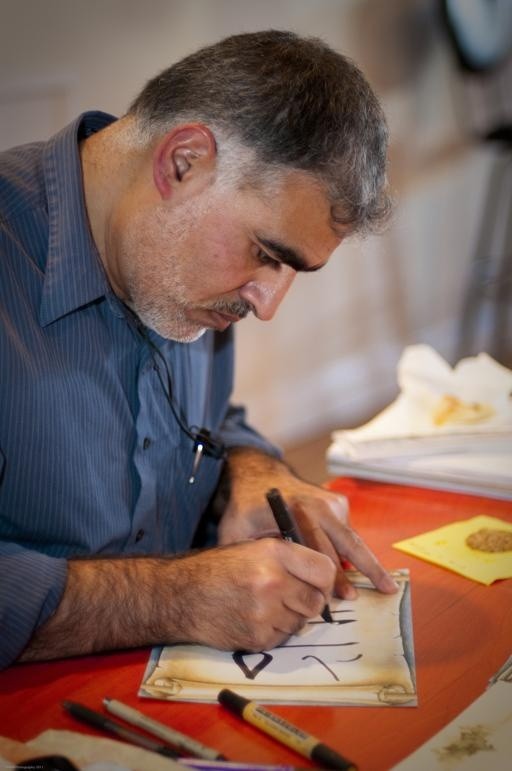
0 30 399 671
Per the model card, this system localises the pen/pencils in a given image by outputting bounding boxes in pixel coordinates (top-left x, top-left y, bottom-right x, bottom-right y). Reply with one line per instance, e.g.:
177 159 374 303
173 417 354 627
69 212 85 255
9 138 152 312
217 691 362 769
63 696 229 767
266 490 336 625
